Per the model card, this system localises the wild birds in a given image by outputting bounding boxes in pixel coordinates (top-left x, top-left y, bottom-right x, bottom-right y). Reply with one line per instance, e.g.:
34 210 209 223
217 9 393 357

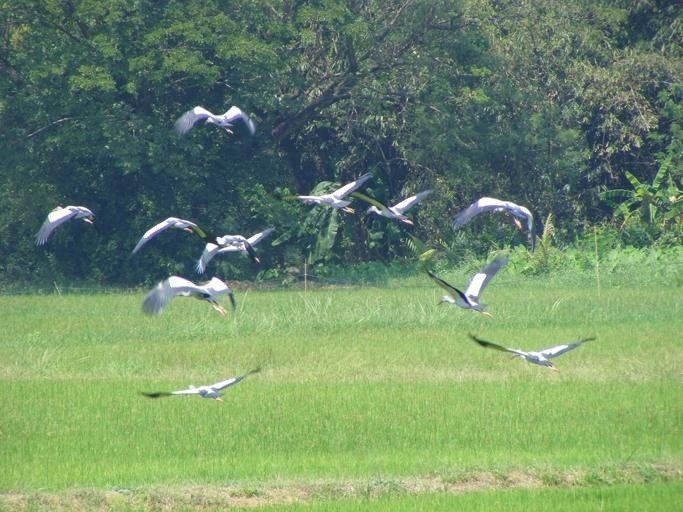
128 216 277 319
422 252 510 320
359 188 434 228
32 203 95 249
467 331 596 367
136 361 265 405
175 103 257 140
282 171 376 215
448 197 536 254
